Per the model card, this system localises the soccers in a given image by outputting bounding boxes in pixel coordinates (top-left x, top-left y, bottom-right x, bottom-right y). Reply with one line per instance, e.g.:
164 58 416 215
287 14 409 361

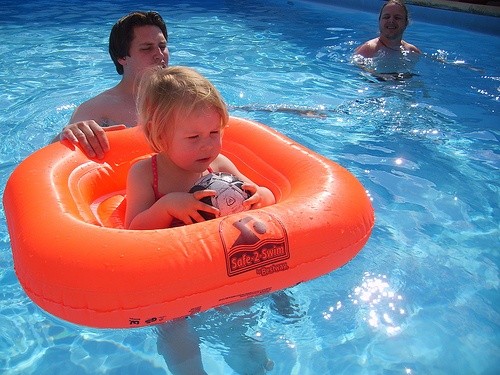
189 172 253 223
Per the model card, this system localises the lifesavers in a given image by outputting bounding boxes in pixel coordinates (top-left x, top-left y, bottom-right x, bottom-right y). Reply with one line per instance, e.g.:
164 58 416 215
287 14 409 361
3 116 374 327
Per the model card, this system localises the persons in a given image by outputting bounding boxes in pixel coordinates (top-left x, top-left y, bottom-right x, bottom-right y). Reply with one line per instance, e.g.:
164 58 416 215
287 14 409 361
49 12 305 375
353 0 483 104
126 65 275 231
